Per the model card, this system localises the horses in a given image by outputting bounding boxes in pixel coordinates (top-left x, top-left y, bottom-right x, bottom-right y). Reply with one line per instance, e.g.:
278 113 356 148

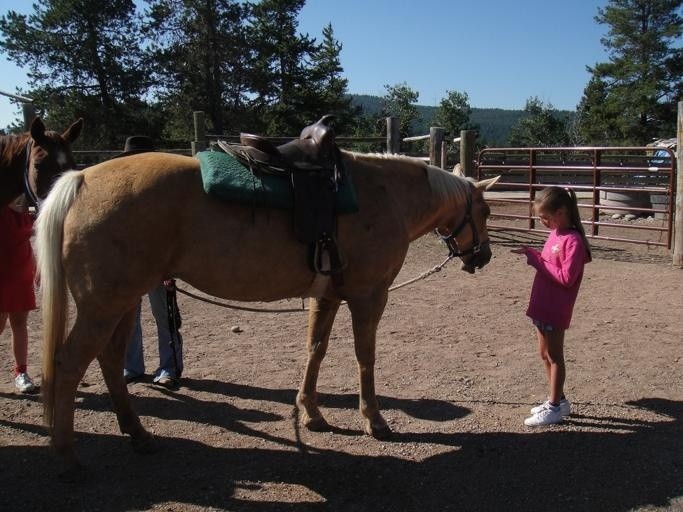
32 148 502 482
0 116 86 212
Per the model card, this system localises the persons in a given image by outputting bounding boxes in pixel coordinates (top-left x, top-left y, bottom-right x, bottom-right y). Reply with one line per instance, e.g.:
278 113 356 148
0 193 41 394
509 185 592 427
108 134 185 389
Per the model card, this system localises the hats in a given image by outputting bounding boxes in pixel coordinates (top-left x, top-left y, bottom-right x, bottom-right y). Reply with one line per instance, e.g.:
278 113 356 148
112 135 163 158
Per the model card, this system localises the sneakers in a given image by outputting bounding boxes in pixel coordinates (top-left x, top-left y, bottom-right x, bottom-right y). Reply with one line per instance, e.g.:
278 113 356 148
159 377 173 387
530 402 570 416
16 374 34 392
524 409 561 426
124 373 142 382
